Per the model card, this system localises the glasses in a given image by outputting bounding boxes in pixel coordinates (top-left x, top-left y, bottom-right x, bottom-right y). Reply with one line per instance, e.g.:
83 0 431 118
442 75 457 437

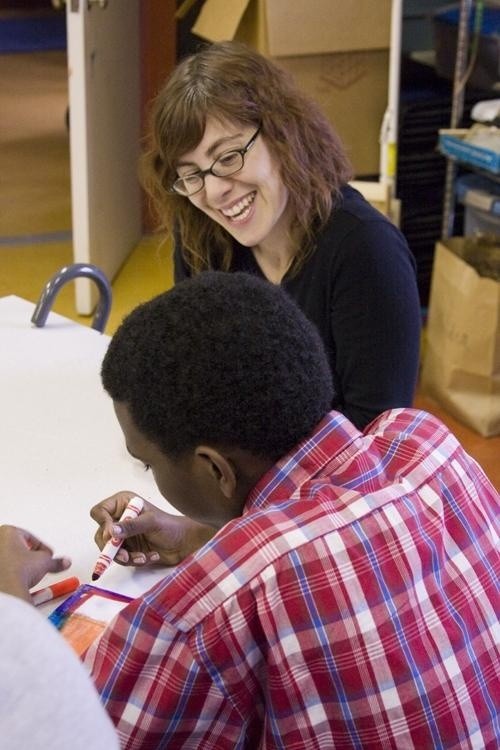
171 118 266 199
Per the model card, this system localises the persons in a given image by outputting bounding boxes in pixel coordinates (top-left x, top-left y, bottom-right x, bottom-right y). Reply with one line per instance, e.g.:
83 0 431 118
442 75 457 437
0 269 500 750
134 39 421 433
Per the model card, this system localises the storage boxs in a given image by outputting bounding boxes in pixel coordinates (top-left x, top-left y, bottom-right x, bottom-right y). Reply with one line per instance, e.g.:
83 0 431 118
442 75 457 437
270 50 389 176
191 0 392 58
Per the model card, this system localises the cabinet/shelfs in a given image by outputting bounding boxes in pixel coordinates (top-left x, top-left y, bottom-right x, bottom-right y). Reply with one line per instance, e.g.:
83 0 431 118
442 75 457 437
438 0 500 243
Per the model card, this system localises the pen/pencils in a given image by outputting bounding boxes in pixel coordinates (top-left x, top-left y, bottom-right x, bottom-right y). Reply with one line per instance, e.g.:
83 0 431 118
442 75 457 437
31 577 79 606
93 497 144 580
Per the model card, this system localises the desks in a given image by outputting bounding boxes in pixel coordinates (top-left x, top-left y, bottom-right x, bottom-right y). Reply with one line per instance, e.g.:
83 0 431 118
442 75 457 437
0 293 181 619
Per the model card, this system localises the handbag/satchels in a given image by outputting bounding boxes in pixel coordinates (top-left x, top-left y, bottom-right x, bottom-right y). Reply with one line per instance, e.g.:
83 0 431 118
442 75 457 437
417 234 500 440
